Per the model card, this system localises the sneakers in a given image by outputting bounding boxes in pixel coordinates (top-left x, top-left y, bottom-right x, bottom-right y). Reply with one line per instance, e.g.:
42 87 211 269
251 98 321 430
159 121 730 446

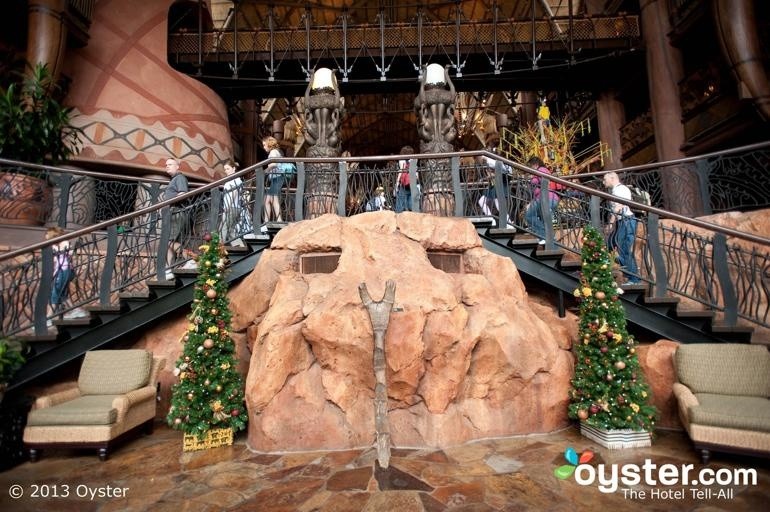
621 281 643 286
32 318 55 333
154 270 175 281
180 259 199 270
507 224 516 230
539 239 546 245
64 309 87 320
484 217 497 226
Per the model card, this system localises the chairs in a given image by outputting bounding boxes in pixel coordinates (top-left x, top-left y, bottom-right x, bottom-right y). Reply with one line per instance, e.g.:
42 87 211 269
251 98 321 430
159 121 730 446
22 349 166 464
671 340 770 464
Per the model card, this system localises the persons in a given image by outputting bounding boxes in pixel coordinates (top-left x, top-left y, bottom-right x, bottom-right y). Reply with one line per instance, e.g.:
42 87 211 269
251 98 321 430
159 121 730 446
474 156 514 229
393 146 418 211
366 187 387 211
218 159 245 247
603 172 640 285
262 136 284 224
165 158 198 281
525 157 560 245
536 102 550 127
31 227 86 330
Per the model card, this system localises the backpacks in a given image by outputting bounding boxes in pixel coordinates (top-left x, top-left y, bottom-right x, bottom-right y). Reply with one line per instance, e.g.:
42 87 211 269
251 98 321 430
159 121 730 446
616 184 652 219
366 195 381 213
549 180 569 198
276 163 297 180
399 161 419 192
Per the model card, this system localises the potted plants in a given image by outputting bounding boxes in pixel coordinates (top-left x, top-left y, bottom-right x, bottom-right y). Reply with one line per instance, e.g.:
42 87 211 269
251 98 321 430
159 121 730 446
1 62 84 226
